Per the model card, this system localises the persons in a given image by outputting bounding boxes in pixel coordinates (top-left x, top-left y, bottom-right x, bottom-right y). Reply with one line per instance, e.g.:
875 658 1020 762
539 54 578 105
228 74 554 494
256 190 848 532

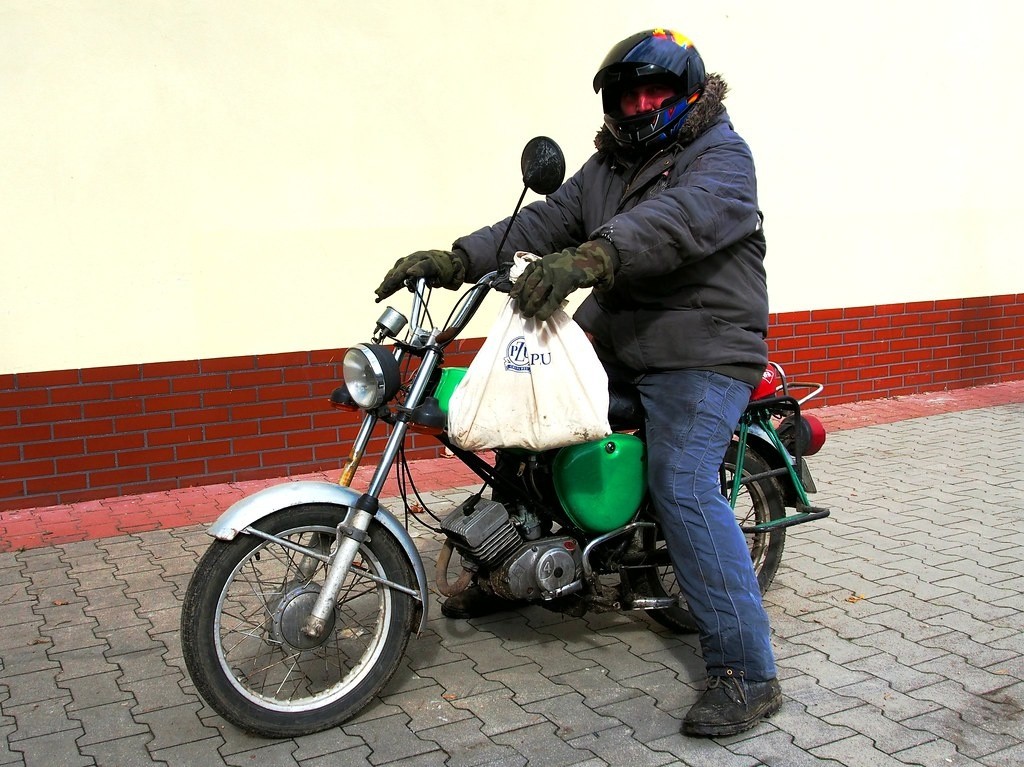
374 29 784 737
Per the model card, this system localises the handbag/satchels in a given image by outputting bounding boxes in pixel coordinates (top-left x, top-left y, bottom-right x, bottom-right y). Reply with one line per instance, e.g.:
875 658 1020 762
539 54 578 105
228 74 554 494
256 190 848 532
448 253 613 453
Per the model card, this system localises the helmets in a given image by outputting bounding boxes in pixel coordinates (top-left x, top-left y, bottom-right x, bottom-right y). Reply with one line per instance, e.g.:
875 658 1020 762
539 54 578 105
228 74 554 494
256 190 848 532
601 29 706 153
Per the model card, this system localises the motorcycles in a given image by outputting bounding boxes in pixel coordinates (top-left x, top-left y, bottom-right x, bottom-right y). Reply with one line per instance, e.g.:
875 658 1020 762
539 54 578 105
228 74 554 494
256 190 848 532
180 136 830 739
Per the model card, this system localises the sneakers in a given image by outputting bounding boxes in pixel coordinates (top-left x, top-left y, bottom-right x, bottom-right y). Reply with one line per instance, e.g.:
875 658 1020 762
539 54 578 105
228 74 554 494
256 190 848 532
441 579 530 620
685 675 782 736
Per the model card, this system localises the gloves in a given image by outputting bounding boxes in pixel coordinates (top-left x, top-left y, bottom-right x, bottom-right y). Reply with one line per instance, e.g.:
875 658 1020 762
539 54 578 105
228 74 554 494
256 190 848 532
374 250 466 300
509 240 615 321
